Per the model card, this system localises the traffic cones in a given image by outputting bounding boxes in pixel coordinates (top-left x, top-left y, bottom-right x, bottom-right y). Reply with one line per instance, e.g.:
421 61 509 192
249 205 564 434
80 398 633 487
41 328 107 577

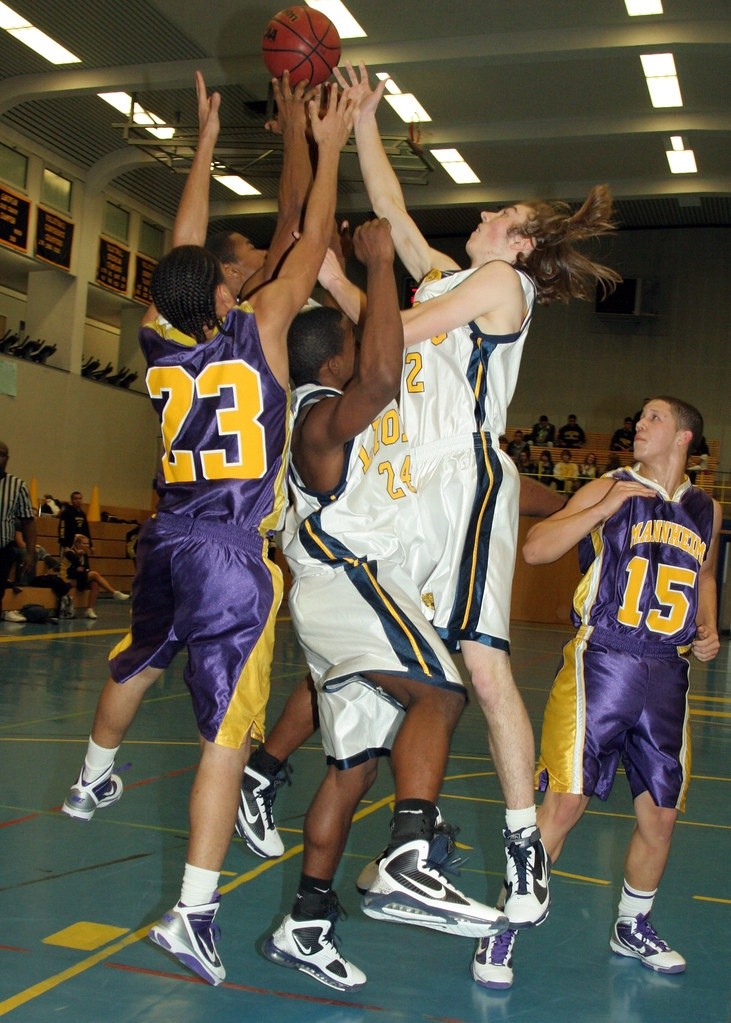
26 477 39 514
84 485 102 522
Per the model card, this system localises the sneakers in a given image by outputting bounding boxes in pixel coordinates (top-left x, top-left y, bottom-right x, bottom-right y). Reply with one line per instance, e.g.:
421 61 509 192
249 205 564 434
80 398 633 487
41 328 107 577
62 762 122 822
470 904 519 990
234 765 285 859
502 825 554 930
261 897 367 993
148 893 227 986
5 610 26 621
360 838 509 938
112 590 130 601
355 806 448 895
85 607 97 618
608 910 687 974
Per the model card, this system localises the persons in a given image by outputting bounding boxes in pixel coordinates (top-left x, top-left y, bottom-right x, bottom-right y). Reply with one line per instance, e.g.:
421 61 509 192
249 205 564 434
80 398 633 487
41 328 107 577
316 60 623 929
55 492 96 556
514 451 538 481
257 218 511 993
551 450 578 492
506 430 530 457
601 454 622 475
528 415 556 447
609 417 633 452
556 414 586 448
60 534 130 619
537 450 554 486
59 71 358 987
683 435 711 486
632 398 650 441
0 439 33 619
577 452 600 479
470 397 722 990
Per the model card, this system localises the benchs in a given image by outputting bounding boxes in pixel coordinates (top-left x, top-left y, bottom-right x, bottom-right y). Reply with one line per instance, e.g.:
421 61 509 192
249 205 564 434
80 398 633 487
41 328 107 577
0 499 153 611
499 429 720 497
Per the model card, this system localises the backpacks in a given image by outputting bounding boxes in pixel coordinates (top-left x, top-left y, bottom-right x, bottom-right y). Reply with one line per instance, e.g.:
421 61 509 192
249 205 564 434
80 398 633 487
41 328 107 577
22 603 51 625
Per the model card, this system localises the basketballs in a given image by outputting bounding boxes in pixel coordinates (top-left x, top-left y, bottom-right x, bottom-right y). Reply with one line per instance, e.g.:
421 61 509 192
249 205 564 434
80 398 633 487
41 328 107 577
261 6 342 87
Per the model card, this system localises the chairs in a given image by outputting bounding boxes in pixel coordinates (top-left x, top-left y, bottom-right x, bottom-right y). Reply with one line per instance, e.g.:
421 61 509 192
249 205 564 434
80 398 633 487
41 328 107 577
81 356 138 387
0 330 57 363
